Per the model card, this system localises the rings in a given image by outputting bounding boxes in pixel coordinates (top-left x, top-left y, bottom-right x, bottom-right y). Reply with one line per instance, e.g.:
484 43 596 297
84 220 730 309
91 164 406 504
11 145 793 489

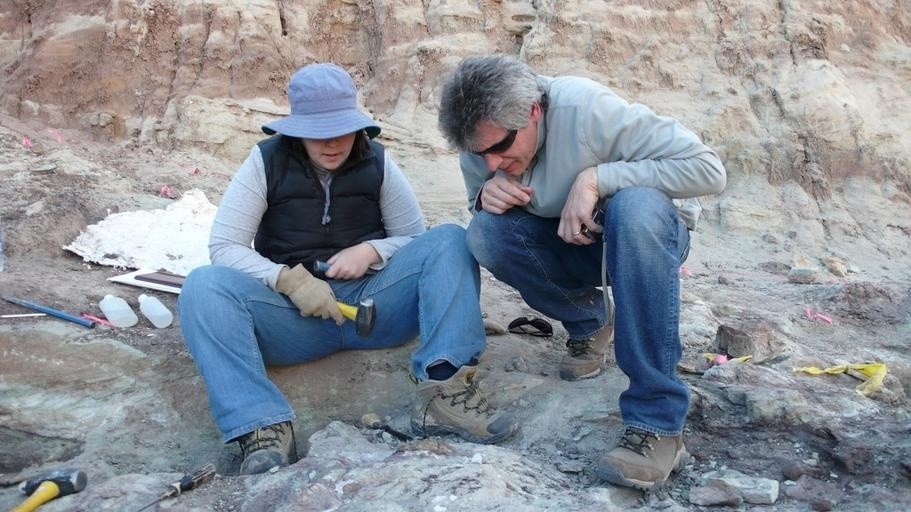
572 231 581 235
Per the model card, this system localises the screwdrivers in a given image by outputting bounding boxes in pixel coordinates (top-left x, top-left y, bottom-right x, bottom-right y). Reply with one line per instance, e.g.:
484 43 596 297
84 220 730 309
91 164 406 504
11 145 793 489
135 462 217 512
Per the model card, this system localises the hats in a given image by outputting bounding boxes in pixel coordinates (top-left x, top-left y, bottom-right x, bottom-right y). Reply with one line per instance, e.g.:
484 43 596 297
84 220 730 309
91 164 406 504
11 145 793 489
260 62 383 143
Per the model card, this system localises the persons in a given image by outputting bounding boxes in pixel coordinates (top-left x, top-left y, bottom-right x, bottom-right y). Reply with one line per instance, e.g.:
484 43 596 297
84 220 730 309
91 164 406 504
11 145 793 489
176 59 524 476
434 51 730 492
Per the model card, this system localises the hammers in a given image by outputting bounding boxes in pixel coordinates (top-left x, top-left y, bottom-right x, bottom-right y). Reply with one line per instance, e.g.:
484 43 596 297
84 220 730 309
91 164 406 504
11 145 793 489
9 469 88 512
336 297 376 337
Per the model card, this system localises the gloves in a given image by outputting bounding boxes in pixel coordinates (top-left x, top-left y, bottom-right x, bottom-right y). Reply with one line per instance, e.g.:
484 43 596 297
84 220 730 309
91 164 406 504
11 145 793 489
276 263 346 328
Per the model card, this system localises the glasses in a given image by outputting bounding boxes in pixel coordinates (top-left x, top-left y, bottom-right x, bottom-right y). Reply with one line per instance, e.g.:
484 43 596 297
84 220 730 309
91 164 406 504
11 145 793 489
507 316 554 338
467 126 519 158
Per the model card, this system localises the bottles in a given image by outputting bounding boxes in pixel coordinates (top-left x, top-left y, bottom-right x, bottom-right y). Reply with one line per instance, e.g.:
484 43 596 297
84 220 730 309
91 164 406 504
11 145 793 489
138 293 174 329
100 293 138 328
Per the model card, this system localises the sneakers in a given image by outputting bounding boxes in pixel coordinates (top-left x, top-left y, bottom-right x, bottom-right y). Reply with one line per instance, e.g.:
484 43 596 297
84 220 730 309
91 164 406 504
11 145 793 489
410 358 519 445
595 425 685 492
239 421 297 477
559 307 614 382
